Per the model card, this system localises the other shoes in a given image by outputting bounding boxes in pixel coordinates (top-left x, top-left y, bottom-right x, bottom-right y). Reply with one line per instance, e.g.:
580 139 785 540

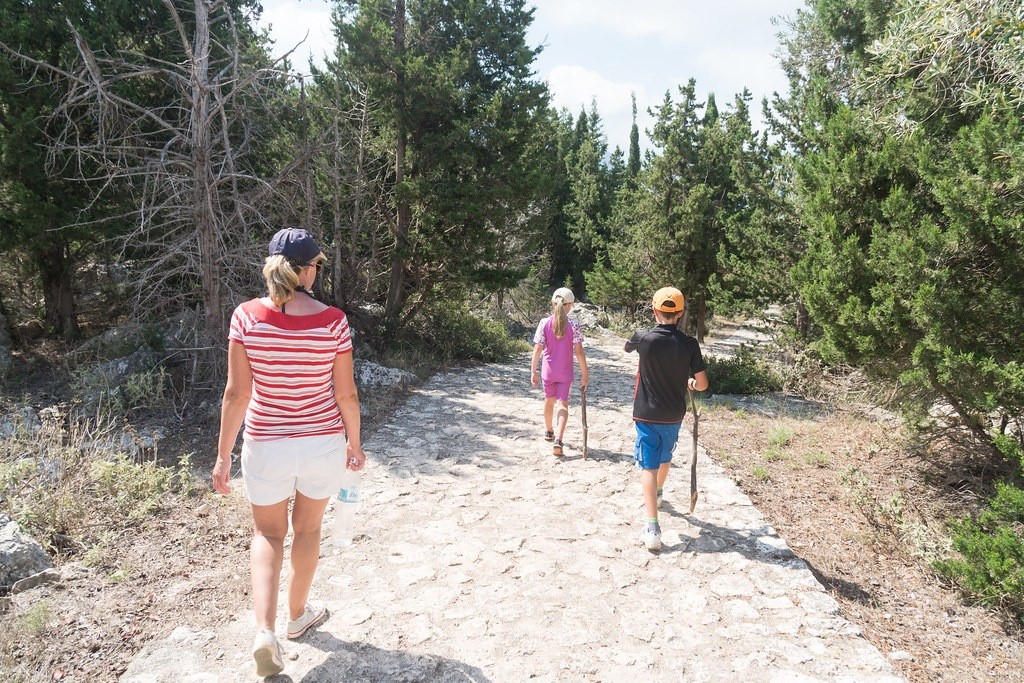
656 495 663 509
639 527 661 550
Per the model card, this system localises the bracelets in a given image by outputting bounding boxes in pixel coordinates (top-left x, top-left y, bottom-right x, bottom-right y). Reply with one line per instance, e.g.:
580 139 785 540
690 380 696 390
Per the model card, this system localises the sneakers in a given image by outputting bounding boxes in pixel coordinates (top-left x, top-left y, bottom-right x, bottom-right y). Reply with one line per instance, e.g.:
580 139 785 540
544 430 554 440
252 629 286 676
285 602 328 639
553 440 564 456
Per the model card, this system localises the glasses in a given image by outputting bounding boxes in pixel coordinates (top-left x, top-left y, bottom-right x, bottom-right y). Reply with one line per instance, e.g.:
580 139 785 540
302 260 323 274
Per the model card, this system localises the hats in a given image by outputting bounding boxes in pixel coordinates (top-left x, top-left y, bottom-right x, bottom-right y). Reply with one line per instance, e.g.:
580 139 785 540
552 287 576 308
652 287 684 312
269 227 328 264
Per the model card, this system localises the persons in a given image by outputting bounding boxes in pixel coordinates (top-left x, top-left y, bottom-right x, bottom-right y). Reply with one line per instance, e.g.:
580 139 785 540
531 287 589 456
212 226 366 678
623 287 709 551
